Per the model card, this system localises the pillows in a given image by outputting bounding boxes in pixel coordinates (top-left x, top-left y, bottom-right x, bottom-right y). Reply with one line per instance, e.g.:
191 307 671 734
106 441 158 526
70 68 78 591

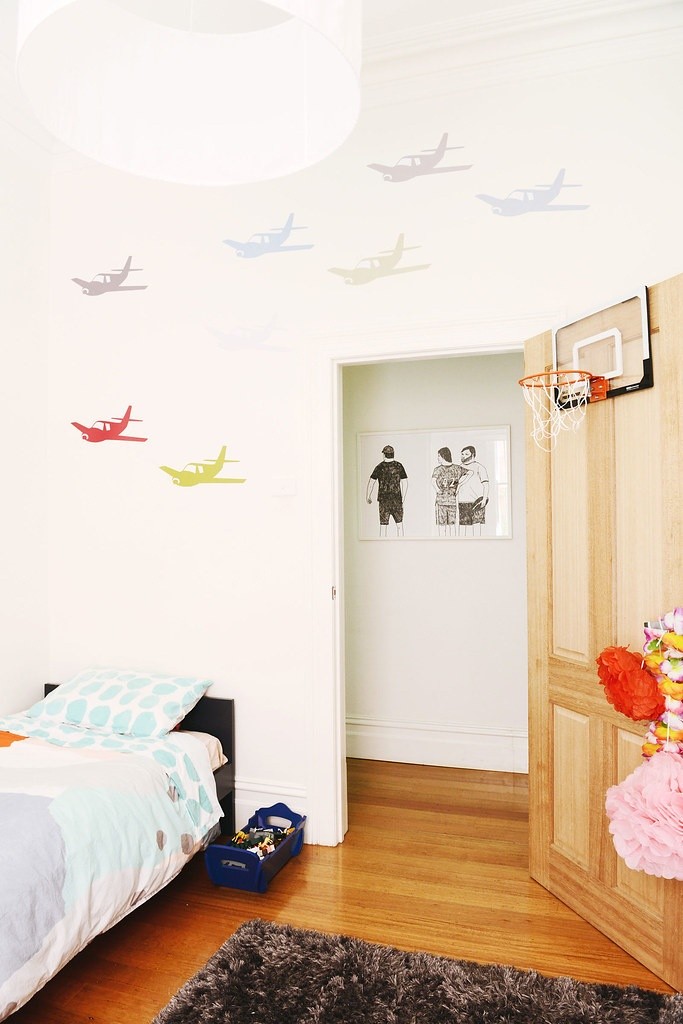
25 666 214 736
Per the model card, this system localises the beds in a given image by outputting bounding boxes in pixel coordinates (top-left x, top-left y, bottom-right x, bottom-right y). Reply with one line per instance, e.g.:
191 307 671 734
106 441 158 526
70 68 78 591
0 685 235 1023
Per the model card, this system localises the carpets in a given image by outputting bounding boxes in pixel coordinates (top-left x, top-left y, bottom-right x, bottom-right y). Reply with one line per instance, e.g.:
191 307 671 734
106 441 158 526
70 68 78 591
151 917 683 1024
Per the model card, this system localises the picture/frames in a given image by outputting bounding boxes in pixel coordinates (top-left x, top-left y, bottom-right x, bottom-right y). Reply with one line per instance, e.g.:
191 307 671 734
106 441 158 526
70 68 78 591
358 425 510 540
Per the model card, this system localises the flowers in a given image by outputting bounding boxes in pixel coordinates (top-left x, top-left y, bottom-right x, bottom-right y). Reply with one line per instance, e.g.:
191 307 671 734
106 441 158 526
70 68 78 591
604 750 683 881
595 643 667 724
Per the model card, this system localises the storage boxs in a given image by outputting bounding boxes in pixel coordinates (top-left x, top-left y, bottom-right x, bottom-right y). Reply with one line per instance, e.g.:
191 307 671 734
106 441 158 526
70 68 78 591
205 801 307 893
